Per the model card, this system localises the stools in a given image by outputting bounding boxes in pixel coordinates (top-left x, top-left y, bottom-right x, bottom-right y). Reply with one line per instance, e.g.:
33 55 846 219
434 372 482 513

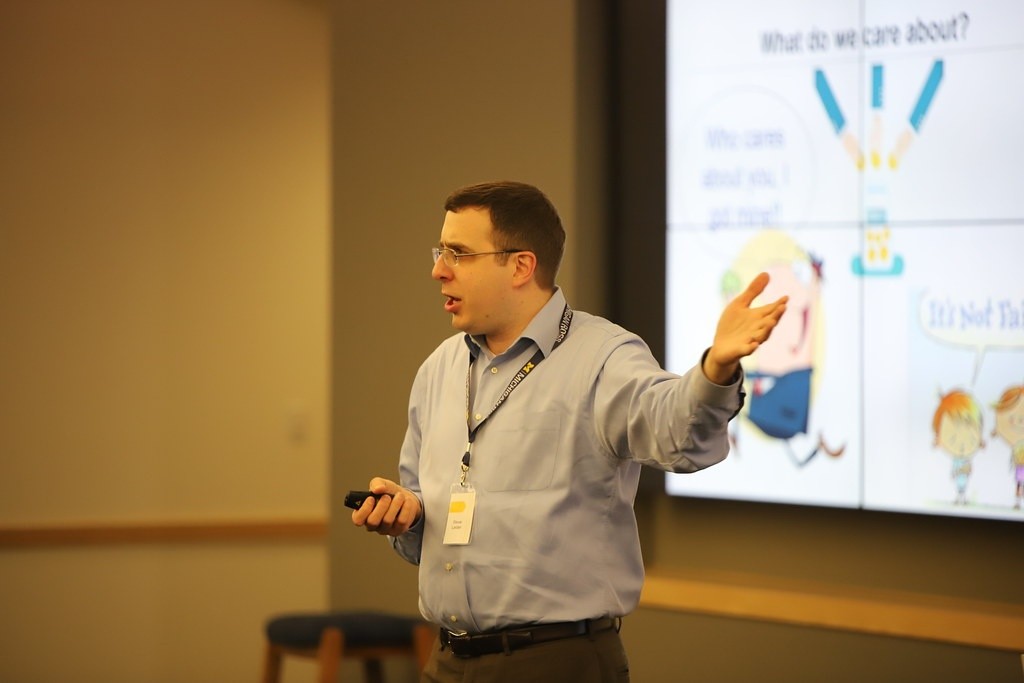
259 607 436 683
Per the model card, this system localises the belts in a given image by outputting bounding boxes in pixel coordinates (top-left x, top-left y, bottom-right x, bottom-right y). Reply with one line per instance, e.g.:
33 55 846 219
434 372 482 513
439 617 616 658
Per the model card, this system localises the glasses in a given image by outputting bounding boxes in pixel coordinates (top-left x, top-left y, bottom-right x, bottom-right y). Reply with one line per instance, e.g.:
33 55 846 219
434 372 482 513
430 246 521 267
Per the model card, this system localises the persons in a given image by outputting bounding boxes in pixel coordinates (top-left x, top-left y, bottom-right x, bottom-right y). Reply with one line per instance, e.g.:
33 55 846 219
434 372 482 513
351 182 791 683
